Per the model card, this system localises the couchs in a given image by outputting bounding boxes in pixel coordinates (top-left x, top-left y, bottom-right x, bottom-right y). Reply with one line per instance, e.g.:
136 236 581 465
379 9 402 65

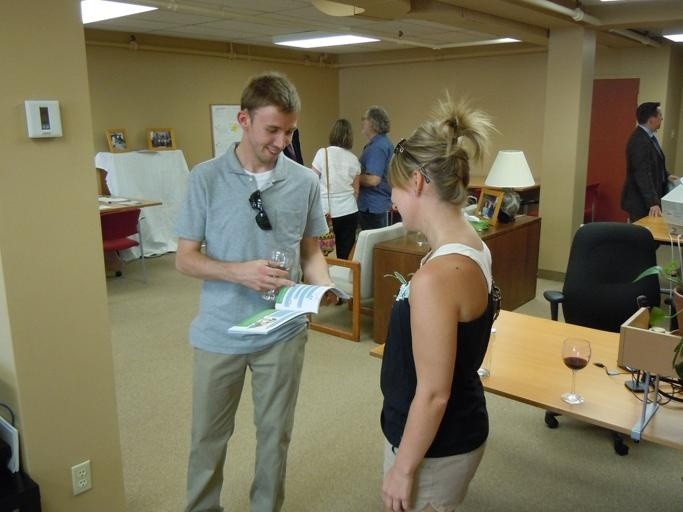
305 221 408 342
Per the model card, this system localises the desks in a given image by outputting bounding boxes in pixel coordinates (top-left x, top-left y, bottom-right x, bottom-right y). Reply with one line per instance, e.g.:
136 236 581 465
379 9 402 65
632 215 683 294
467 175 540 216
99 195 163 277
586 180 602 223
373 214 542 345
369 307 683 451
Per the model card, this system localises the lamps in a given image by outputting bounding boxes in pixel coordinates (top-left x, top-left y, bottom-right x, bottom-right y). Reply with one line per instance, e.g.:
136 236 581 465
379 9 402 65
485 149 536 218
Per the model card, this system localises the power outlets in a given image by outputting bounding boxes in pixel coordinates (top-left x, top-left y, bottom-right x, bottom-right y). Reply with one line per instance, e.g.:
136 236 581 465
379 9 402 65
71 459 92 496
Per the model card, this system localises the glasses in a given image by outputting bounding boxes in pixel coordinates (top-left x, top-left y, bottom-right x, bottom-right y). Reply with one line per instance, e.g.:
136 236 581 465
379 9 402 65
249 188 273 231
393 138 432 183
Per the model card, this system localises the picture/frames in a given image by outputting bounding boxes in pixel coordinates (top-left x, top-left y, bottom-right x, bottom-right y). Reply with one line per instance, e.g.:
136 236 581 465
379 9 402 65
146 127 177 151
474 186 504 227
104 128 131 153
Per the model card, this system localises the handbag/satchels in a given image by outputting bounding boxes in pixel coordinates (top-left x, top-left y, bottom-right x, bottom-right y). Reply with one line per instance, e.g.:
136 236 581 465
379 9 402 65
318 147 336 256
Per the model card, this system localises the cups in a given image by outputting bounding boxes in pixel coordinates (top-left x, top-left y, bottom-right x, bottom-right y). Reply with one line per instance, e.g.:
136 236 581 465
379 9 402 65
262 247 296 301
561 339 591 405
477 328 496 378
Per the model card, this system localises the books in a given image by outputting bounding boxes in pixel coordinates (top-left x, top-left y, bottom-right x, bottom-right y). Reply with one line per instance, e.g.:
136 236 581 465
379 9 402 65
226 282 351 335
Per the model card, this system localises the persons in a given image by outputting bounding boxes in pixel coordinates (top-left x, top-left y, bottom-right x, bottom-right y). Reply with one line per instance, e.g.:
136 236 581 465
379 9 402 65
481 201 488 215
360 106 395 230
619 101 679 224
310 119 362 260
379 100 503 511
174 71 341 512
488 199 493 217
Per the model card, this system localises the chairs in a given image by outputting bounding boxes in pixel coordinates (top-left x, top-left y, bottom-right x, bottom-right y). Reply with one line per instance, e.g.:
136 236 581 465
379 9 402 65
542 222 679 456
100 208 147 283
